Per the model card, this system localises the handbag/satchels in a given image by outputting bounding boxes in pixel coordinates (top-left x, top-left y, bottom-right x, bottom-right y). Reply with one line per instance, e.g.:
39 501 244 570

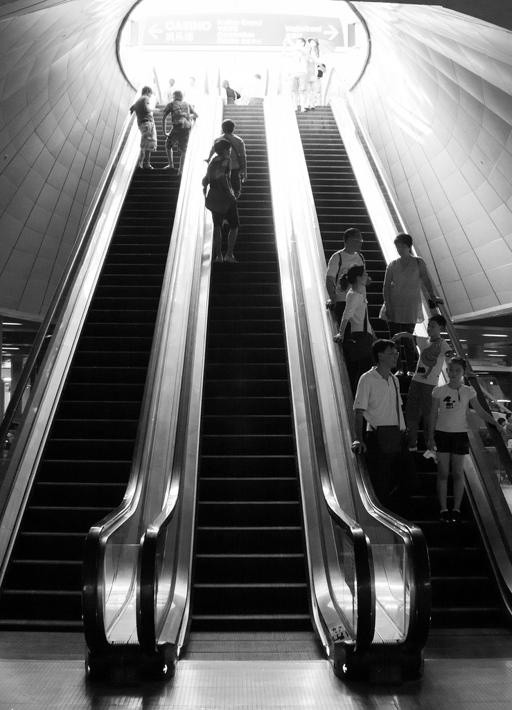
349 334 371 363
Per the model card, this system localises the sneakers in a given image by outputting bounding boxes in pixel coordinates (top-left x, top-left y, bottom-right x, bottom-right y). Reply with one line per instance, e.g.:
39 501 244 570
440 509 461 523
205 186 234 214
138 161 174 172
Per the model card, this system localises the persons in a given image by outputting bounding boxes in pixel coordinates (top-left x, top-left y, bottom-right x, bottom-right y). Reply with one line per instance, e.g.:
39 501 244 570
202 138 243 264
222 79 242 105
393 316 455 451
167 78 177 105
428 357 508 526
350 342 415 520
307 37 320 112
158 92 200 177
377 235 444 379
131 86 159 171
326 227 373 333
498 417 507 428
505 413 512 433
332 266 375 374
293 37 308 111
204 119 248 202
